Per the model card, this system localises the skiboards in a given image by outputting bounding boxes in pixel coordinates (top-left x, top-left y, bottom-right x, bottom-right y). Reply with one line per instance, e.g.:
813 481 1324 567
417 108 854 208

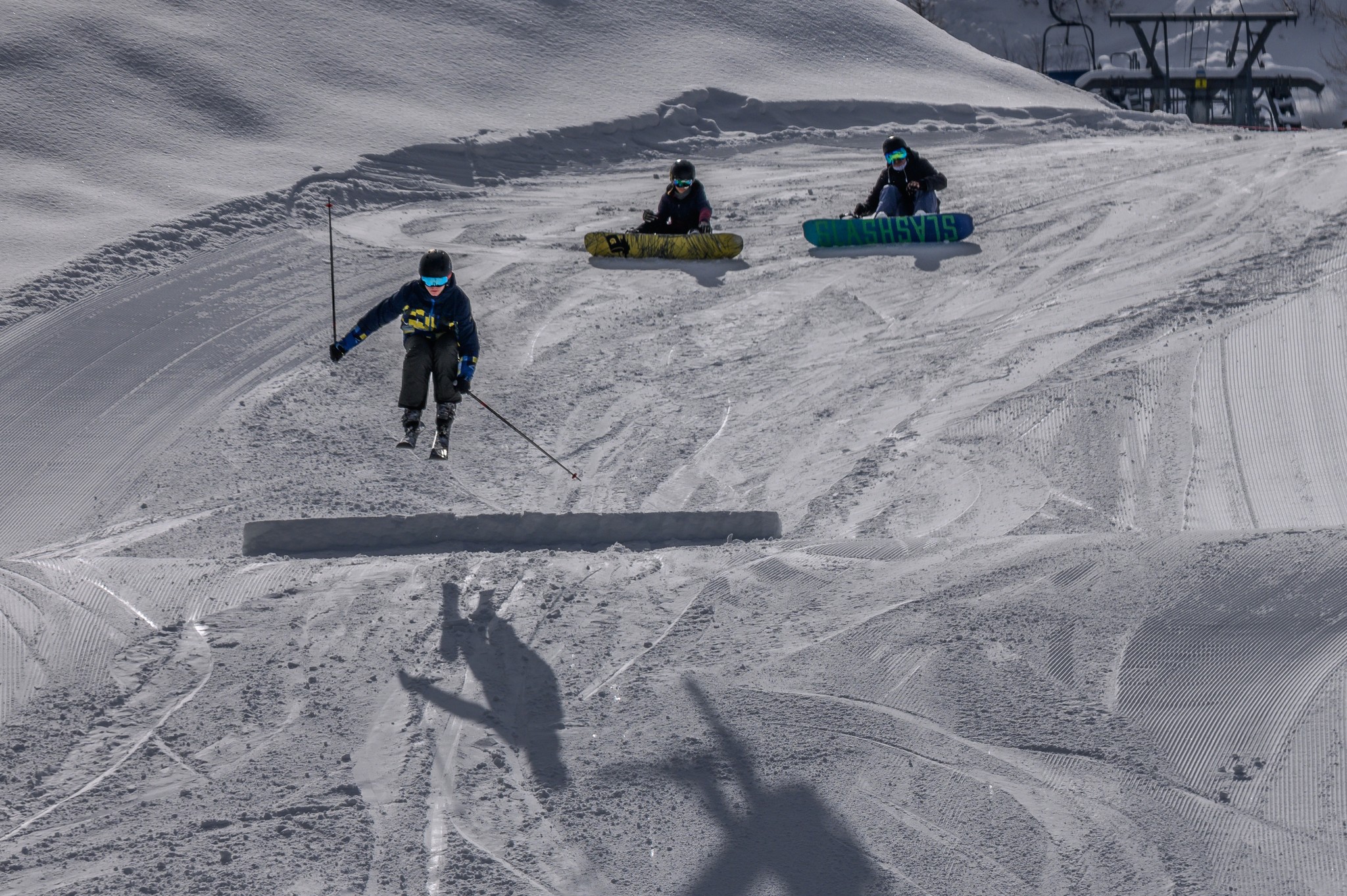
395 421 449 461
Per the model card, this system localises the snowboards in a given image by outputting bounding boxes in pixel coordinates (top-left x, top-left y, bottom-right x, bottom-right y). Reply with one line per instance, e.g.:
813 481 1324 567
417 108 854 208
801 212 975 247
584 231 744 259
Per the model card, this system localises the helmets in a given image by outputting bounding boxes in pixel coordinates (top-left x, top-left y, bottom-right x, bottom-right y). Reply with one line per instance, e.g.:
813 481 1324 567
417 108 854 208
883 136 908 155
670 160 695 183
419 249 452 279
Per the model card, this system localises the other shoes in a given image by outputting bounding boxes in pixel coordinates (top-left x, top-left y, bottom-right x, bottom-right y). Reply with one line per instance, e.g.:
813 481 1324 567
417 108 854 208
435 402 457 427
914 209 926 216
625 227 636 233
690 229 701 235
875 211 888 219
403 408 421 432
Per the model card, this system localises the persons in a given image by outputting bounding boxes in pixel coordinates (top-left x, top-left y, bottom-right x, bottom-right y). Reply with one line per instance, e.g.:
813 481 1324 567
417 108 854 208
854 136 948 218
330 248 479 443
629 159 712 235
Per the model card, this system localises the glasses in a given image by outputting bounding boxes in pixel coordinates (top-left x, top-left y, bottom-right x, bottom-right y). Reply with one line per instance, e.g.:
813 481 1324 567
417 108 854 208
673 178 692 187
885 147 907 164
421 275 448 287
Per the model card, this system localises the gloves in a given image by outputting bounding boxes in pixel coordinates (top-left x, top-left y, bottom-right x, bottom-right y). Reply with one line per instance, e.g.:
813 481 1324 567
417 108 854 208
698 220 713 234
908 181 920 191
453 380 470 395
642 209 658 223
329 343 349 362
